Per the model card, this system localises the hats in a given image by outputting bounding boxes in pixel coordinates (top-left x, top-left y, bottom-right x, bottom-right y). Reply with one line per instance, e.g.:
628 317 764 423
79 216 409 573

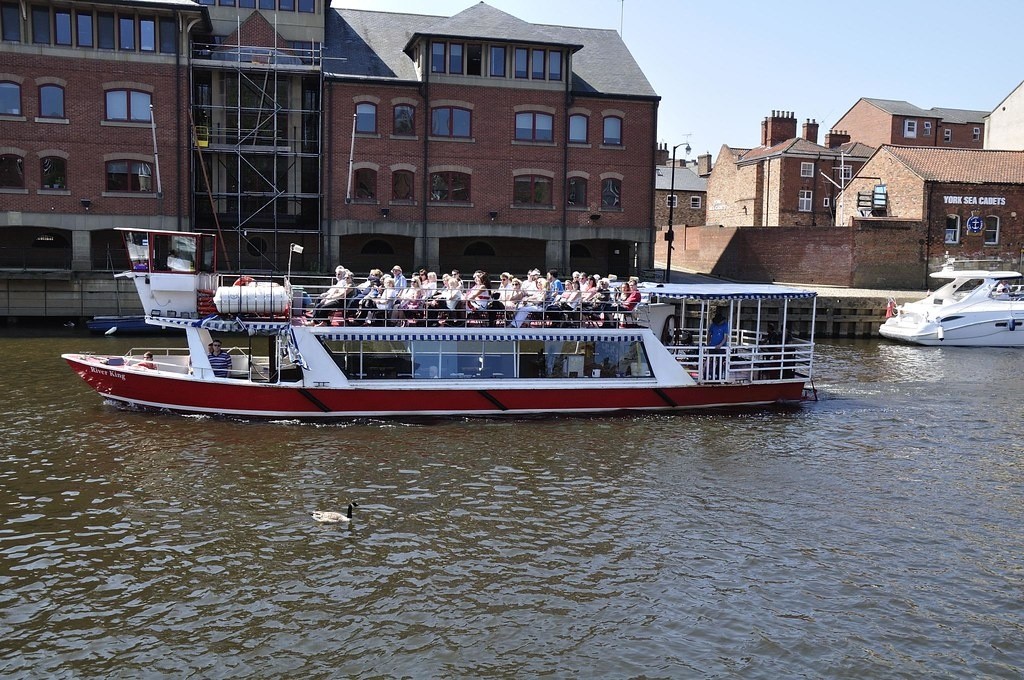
391 266 402 273
712 313 726 323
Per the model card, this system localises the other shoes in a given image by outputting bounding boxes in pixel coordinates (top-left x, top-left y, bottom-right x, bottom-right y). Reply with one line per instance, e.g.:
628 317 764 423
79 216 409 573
305 320 315 325
316 321 327 326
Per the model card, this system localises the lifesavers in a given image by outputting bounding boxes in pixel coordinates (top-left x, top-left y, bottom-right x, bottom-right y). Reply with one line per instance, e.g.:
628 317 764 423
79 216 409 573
197 307 216 311
197 297 213 302
233 276 256 287
198 311 219 315
197 289 215 294
197 302 212 307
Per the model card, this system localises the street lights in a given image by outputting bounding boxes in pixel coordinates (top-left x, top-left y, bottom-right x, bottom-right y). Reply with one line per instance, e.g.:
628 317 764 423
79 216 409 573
664 141 691 282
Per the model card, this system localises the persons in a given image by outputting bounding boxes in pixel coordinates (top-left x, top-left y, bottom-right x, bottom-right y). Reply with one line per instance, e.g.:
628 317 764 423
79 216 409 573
547 356 568 377
705 310 730 385
306 265 642 327
759 320 793 371
537 348 546 376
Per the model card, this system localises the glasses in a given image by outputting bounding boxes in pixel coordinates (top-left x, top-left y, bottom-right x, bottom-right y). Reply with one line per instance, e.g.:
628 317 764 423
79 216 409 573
575 277 579 278
512 284 518 285
452 274 457 276
214 344 220 347
501 279 506 281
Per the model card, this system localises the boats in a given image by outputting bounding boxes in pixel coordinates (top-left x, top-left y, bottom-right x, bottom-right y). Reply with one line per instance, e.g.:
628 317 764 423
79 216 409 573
86 314 179 337
60 226 819 419
878 253 1024 349
